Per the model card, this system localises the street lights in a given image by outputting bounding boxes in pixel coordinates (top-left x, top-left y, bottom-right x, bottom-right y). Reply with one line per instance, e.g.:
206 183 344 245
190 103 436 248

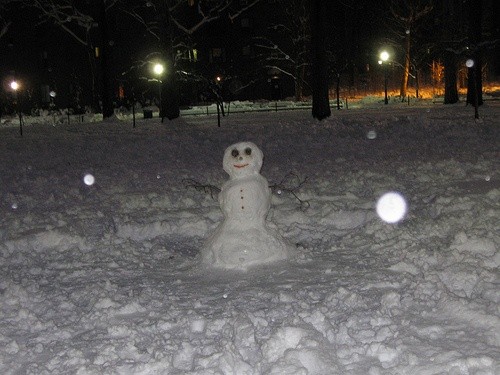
11 80 22 114
380 50 389 103
152 64 167 121
